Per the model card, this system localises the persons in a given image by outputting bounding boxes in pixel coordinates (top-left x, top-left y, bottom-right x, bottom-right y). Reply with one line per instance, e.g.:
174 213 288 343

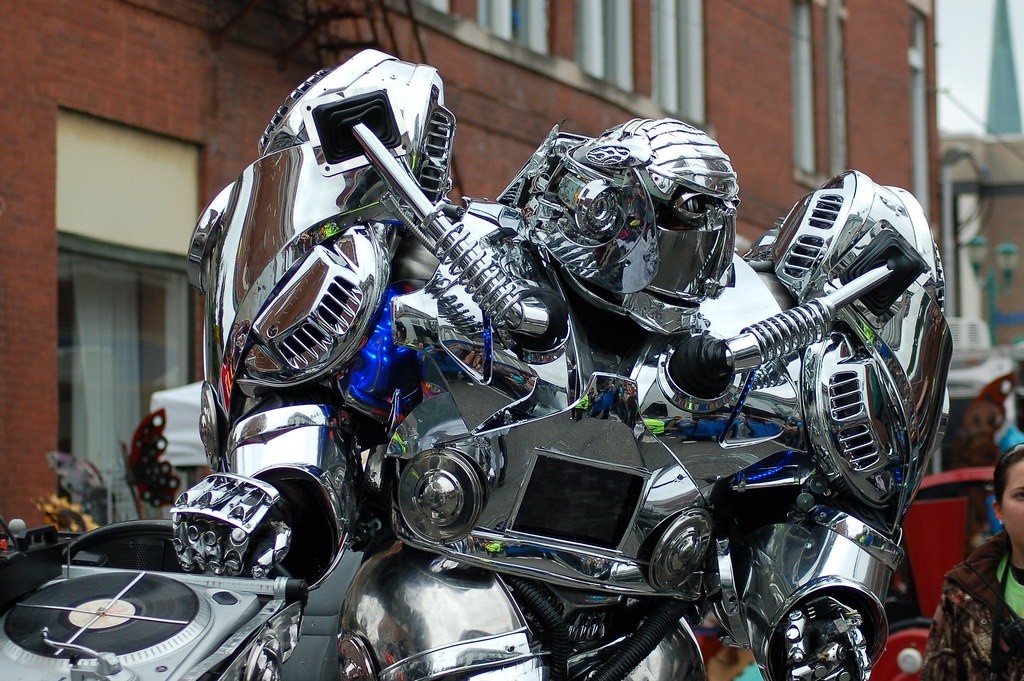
923 440 1024 681
1 48 956 681
